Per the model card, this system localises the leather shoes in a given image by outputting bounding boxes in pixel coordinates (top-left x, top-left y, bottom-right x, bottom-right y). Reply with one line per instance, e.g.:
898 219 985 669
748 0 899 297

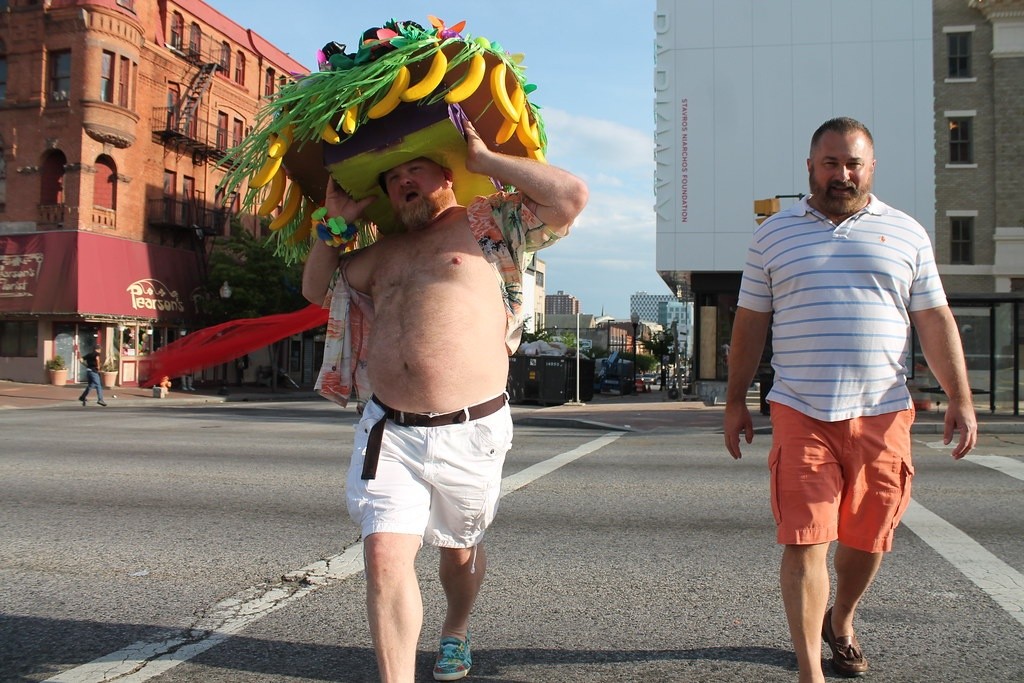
822 605 868 676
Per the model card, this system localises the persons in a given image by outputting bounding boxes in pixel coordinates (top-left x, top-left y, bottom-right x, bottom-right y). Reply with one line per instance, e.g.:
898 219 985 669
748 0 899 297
724 118 979 683
181 375 196 391
79 345 107 406
300 120 588 683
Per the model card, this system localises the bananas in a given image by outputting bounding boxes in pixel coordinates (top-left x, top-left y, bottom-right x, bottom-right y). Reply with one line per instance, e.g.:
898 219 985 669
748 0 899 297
246 43 546 252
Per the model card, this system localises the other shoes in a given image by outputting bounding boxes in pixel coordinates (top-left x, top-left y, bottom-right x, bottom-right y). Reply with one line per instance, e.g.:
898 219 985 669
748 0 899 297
97 399 107 406
79 397 87 401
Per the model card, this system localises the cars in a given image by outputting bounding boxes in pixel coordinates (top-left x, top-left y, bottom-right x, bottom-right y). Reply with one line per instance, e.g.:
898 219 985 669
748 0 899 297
675 366 689 377
642 373 657 385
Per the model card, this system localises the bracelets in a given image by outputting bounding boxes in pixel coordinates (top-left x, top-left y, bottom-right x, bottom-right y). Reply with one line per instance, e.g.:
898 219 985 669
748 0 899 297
310 207 358 248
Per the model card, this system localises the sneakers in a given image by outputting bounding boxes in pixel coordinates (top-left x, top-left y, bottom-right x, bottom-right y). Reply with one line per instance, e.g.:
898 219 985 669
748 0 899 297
433 630 473 680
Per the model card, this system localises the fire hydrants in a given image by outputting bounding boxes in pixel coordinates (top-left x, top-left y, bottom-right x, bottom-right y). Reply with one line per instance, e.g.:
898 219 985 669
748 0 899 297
160 375 172 394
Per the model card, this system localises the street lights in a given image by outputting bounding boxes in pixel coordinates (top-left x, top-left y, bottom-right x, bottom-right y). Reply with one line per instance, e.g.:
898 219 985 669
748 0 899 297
630 311 641 394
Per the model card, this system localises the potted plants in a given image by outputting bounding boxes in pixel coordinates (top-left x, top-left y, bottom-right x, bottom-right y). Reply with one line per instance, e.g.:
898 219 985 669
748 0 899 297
47 354 69 386
98 363 118 387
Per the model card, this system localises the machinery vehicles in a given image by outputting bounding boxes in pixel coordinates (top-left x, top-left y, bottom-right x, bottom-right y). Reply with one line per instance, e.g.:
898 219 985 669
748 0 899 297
596 350 636 395
